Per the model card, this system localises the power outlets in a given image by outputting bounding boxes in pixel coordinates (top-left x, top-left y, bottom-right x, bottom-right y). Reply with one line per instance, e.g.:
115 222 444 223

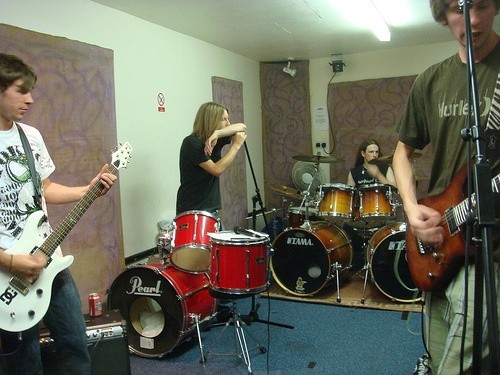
315 142 326 152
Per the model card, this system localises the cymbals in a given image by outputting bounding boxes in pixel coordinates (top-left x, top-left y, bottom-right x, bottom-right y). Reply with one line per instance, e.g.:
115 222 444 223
271 184 307 201
292 154 346 163
367 152 423 164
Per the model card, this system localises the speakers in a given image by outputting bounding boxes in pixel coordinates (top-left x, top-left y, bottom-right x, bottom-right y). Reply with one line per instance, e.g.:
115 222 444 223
332 60 343 72
39 315 131 375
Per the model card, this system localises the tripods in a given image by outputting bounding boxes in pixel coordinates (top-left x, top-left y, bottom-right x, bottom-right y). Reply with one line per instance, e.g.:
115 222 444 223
200 142 294 375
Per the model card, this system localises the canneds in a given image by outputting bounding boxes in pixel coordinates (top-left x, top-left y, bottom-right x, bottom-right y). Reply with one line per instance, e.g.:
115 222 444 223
88 293 102 317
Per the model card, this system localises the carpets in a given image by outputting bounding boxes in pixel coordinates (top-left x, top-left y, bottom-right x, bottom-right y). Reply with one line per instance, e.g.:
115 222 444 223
259 281 424 312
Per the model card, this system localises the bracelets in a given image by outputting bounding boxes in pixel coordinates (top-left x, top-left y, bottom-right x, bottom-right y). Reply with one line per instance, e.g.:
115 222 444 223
8 255 13 272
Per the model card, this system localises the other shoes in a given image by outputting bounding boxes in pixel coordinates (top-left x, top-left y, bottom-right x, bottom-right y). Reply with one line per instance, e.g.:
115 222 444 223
216 313 251 324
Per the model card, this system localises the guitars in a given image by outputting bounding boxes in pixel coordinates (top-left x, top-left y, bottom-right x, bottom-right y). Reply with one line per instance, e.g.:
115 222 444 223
405 159 500 292
0 141 133 333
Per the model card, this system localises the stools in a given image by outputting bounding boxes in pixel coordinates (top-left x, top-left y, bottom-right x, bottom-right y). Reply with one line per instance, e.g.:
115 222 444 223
342 232 371 282
201 287 267 375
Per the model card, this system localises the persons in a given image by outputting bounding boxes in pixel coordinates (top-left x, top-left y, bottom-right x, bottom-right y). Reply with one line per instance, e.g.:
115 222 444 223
176 102 247 322
392 0 500 375
343 139 396 265
0 53 118 375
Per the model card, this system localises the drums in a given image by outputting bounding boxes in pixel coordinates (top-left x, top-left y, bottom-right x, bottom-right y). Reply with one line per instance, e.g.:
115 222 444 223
287 206 318 229
358 183 399 223
169 209 222 275
106 264 220 359
367 221 424 303
269 220 354 297
315 182 360 222
208 230 271 295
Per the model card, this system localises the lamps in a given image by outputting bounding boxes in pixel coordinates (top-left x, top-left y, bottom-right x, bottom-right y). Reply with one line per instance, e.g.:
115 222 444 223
283 58 296 77
355 0 391 41
